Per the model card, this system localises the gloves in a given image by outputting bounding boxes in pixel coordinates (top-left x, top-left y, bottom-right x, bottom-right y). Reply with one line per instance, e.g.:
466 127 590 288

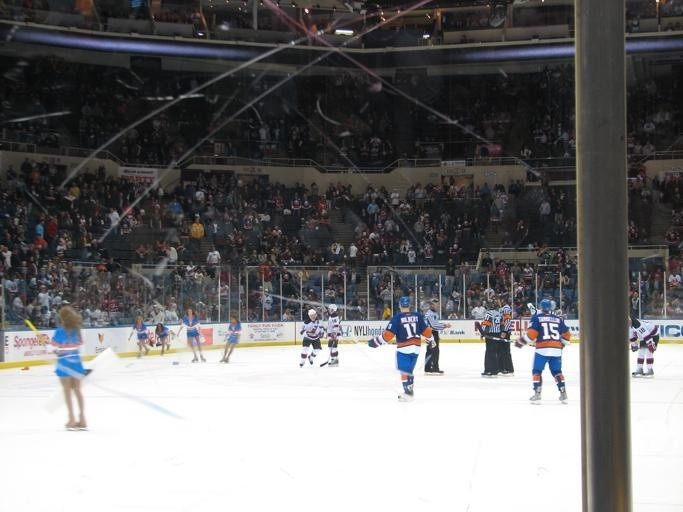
425 335 437 348
368 337 381 348
631 342 638 352
515 337 527 348
646 338 656 353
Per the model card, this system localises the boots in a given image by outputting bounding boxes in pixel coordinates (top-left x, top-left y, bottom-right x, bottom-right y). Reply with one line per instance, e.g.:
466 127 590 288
559 387 567 401
398 376 414 398
529 386 541 401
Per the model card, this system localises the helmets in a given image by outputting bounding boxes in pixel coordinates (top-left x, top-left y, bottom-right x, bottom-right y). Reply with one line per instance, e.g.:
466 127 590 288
484 292 511 311
328 304 338 315
308 309 318 321
540 299 556 311
399 297 410 307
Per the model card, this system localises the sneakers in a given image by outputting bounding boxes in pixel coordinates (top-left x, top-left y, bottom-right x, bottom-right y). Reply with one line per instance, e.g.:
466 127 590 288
300 356 338 366
632 368 654 375
137 349 150 358
425 368 443 373
481 369 514 375
65 422 87 428
193 356 229 363
160 344 170 355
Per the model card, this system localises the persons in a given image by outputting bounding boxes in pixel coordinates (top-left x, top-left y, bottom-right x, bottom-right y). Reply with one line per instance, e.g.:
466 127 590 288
42 305 90 428
369 296 433 402
0 58 683 379
515 299 572 404
191 8 201 39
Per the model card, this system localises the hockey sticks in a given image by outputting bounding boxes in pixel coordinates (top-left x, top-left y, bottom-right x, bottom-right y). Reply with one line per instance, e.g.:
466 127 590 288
476 322 521 343
320 342 333 367
